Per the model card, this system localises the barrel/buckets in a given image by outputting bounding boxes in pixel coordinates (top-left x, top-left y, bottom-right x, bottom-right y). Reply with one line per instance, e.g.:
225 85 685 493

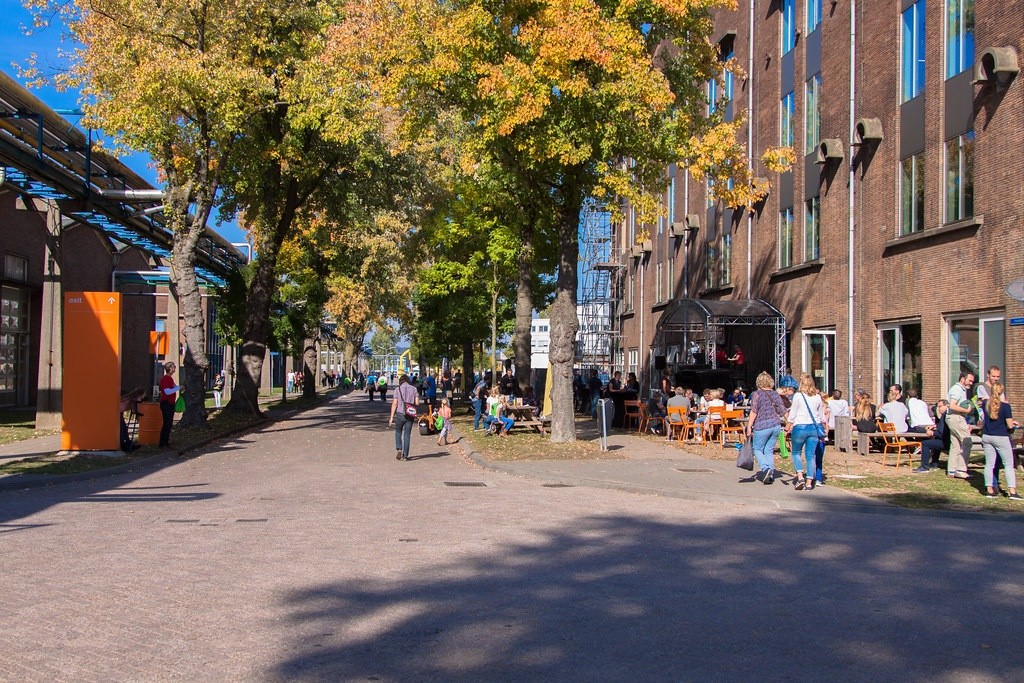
139 403 163 447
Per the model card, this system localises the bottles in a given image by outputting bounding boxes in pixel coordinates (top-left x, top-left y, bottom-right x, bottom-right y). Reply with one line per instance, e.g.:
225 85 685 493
513 397 517 406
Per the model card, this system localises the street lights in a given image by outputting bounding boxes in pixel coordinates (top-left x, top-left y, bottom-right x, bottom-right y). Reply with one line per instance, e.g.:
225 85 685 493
378 345 396 378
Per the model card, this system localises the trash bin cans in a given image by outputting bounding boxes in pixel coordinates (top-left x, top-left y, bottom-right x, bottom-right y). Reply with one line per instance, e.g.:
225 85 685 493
597 398 615 450
579 388 589 412
136 403 163 445
611 388 638 427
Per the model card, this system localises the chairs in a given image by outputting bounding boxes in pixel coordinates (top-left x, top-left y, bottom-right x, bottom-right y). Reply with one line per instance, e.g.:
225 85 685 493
877 420 921 469
624 399 747 451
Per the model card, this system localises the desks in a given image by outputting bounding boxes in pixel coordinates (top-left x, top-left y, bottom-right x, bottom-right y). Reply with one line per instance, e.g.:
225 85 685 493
690 410 707 413
732 419 748 423
498 407 543 436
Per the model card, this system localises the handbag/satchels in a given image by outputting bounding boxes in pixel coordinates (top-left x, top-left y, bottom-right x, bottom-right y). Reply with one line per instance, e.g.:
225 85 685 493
778 430 789 459
816 425 826 438
404 402 417 417
736 444 754 471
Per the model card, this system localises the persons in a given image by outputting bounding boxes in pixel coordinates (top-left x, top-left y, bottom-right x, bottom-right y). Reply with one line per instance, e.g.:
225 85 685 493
366 370 394 402
945 370 974 479
977 366 1024 500
321 371 364 390
388 372 420 461
693 342 745 367
420 369 540 446
574 368 751 443
747 371 788 484
158 362 185 451
785 373 828 491
213 373 222 406
287 369 304 394
120 412 141 453
825 384 951 473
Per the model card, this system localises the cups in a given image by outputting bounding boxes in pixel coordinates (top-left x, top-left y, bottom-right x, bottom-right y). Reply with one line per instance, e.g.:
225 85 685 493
702 408 706 412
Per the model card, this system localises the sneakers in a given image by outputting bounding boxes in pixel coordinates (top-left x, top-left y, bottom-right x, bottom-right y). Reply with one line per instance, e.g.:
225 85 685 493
929 462 940 469
985 491 998 498
1008 492 1024 500
795 478 806 490
912 466 930 473
805 485 813 491
762 468 771 483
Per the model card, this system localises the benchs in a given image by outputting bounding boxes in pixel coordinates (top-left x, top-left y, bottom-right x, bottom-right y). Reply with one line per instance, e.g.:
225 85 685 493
483 415 551 426
857 432 932 456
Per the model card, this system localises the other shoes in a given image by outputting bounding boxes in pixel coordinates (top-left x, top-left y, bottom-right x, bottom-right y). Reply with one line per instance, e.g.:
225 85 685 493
954 470 974 479
947 471 955 478
445 442 450 445
437 442 441 446
128 440 141 454
396 451 402 460
403 458 408 461
160 445 171 451
815 480 824 487
